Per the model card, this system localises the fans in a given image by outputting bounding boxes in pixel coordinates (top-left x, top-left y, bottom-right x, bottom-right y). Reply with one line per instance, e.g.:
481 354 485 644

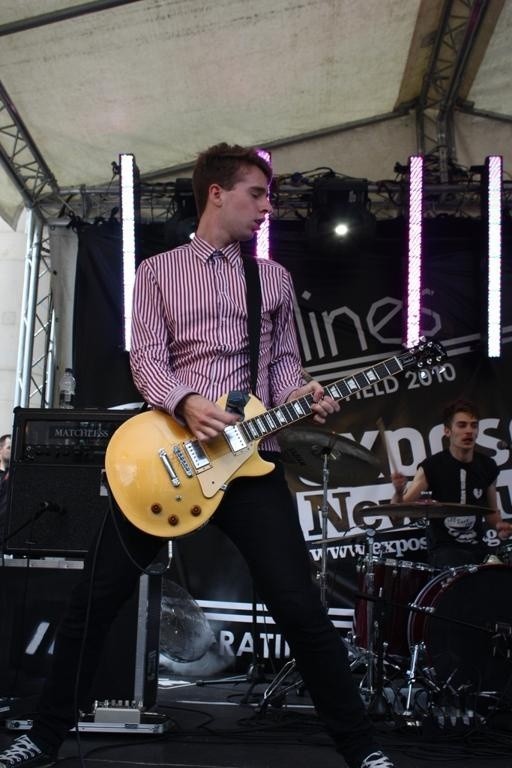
158 578 219 665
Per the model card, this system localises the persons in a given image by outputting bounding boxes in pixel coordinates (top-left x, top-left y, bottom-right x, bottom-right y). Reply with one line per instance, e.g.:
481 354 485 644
391 401 512 581
0 142 400 767
0 434 13 506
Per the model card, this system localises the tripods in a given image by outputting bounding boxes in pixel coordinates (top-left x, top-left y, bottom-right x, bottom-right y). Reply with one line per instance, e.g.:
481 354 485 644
197 470 503 731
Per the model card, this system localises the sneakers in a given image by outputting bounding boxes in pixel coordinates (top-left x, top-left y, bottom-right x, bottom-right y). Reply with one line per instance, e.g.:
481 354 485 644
358 746 396 767
0 730 61 768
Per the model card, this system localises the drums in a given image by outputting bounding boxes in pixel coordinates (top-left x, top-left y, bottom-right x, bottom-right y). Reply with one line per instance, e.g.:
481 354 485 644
408 563 512 717
352 553 454 669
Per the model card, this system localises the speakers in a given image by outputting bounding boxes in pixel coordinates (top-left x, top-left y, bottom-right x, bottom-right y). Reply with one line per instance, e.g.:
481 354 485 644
4 406 144 556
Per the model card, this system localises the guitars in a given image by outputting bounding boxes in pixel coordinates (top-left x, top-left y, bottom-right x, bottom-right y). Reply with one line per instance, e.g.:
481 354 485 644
105 337 448 537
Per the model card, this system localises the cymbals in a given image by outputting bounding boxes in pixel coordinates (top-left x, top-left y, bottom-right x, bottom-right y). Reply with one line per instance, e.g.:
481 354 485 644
276 426 383 485
354 499 494 519
